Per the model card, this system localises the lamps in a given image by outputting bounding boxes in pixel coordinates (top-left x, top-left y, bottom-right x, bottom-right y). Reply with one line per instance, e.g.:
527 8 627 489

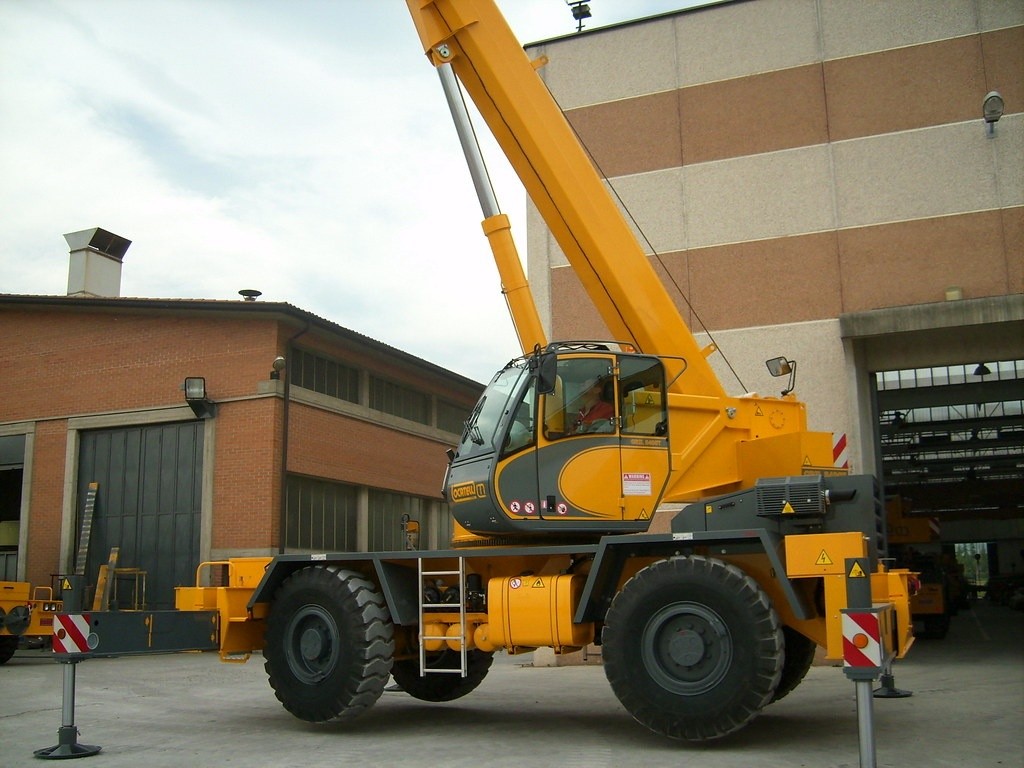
975 363 991 375
981 91 1004 138
184 377 214 418
565 0 592 31
879 412 907 426
269 356 287 380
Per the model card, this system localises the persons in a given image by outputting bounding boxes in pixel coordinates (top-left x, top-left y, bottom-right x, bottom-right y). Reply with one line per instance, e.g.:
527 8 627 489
569 377 614 436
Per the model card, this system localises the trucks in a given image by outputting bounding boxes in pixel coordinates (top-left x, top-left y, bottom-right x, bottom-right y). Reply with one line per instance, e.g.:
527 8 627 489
0 581 63 666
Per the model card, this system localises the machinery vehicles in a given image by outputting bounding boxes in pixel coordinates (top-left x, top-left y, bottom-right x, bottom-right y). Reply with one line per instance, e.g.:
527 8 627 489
32 0 920 768
877 494 970 639
